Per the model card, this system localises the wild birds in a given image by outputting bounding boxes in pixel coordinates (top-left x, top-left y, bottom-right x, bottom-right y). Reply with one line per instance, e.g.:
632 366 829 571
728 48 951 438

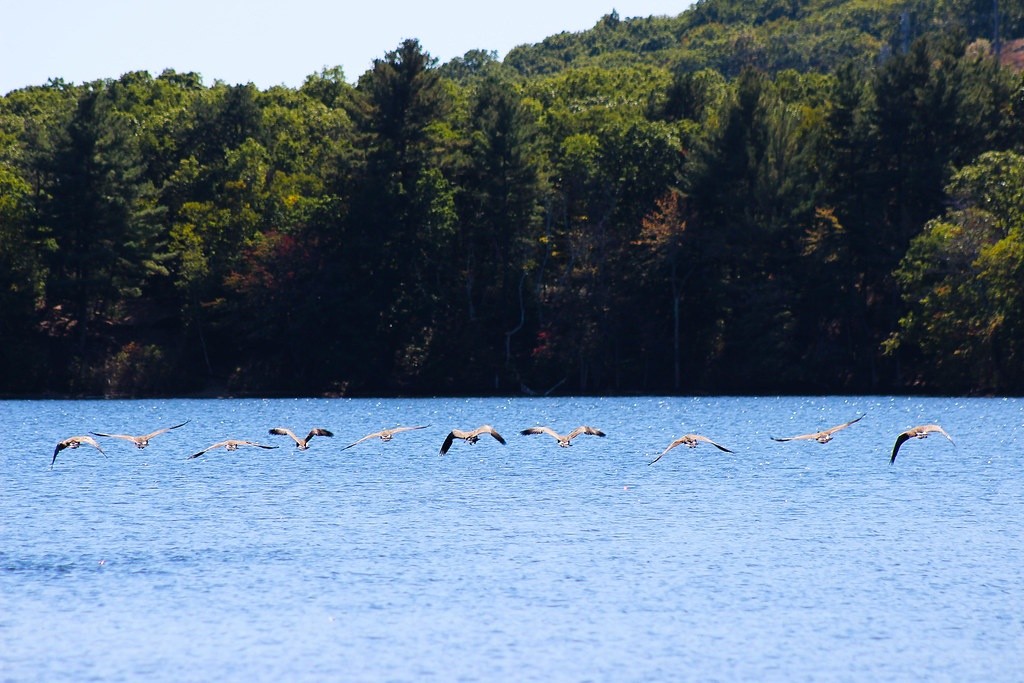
187 440 279 461
519 425 607 448
88 419 192 450
769 412 868 445
648 434 734 468
268 427 334 450
887 425 956 467
439 424 506 457
340 423 431 453
49 436 109 466
